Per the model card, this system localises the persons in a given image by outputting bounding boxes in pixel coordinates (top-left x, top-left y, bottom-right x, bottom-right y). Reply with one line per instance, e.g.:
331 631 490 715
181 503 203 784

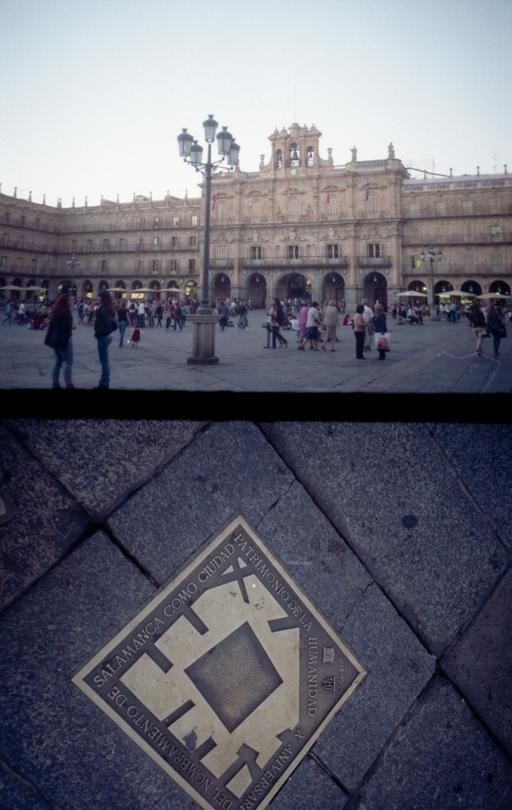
42 291 75 388
94 291 117 388
3 285 511 362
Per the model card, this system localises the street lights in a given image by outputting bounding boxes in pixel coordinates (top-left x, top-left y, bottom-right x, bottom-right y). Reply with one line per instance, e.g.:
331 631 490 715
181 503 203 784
176 112 242 366
419 243 445 322
64 251 83 296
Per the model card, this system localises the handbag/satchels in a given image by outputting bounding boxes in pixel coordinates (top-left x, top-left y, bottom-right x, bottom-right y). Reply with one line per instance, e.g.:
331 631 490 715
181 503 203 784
45 333 68 350
499 324 506 336
111 321 117 330
